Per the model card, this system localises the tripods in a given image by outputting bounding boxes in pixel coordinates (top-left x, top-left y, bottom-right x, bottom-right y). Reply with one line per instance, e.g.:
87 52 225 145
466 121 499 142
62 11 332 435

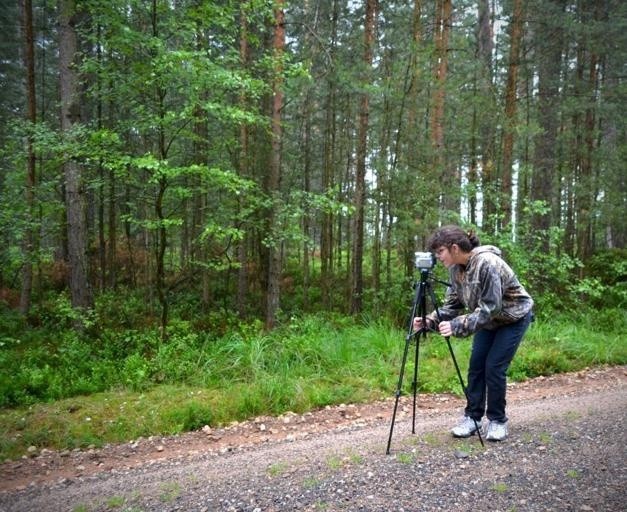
386 270 484 456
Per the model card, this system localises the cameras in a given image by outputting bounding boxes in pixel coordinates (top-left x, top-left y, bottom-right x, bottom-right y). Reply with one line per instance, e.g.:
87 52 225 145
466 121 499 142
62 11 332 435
413 251 437 269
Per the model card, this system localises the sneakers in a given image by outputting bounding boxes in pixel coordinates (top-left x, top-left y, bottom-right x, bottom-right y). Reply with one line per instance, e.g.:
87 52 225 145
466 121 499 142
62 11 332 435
485 417 509 441
450 417 484 438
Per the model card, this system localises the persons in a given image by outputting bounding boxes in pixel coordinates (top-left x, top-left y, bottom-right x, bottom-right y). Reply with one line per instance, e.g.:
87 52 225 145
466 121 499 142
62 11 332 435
413 224 535 442
